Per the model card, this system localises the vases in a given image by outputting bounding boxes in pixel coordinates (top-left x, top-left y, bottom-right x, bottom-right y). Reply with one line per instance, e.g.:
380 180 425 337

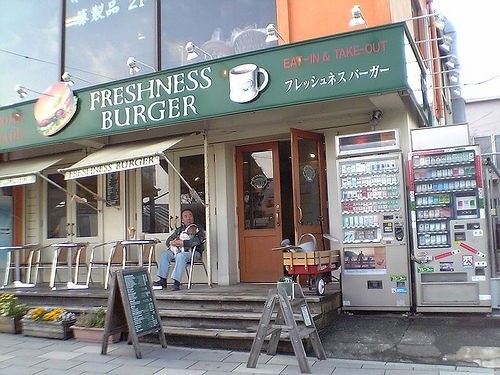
0 316 22 334
20 318 77 341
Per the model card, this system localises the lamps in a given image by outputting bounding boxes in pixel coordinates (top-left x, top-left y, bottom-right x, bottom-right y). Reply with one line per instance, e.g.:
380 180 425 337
423 54 456 68
185 41 214 60
127 57 156 74
265 24 286 44
430 69 460 82
349 5 368 28
406 12 447 30
15 84 44 98
369 111 382 126
434 85 461 96
62 72 94 87
414 35 453 53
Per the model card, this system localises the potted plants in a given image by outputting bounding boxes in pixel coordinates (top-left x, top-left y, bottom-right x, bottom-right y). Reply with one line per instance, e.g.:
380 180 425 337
71 306 122 344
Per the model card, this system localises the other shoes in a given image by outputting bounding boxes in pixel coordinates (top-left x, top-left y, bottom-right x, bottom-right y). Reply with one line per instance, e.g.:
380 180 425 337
170 284 180 291
153 281 167 288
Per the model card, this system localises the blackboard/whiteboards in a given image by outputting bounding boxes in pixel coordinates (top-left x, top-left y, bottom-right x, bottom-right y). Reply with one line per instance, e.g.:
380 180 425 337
104 267 163 337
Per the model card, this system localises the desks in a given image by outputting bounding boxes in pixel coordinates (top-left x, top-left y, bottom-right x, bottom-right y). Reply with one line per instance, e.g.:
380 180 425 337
51 243 88 289
121 239 154 270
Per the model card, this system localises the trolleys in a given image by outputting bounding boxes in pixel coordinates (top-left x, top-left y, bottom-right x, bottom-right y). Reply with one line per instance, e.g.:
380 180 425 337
271 239 340 296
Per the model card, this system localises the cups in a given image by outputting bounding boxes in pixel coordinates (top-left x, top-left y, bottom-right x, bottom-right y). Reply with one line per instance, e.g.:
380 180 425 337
140 233 145 240
128 227 136 239
228 64 269 102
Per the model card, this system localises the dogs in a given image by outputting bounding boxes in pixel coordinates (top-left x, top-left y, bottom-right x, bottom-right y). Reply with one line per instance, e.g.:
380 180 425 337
168 224 199 257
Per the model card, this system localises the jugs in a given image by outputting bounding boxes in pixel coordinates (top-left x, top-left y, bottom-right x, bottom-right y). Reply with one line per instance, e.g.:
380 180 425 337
281 233 317 252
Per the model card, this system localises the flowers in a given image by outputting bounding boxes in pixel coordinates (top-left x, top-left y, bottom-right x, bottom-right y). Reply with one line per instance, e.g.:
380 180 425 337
21 307 76 323
0 294 27 317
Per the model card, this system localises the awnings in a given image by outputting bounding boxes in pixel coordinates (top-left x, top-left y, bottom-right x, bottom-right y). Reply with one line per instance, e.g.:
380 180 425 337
57 132 198 179
0 148 86 188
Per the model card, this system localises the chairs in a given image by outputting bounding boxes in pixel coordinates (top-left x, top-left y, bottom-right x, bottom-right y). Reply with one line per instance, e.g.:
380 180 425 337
8 238 209 289
201 30 274 60
0 246 31 290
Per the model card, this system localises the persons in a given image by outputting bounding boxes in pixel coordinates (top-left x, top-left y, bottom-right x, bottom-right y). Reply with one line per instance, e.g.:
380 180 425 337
348 251 365 268
152 209 206 291
